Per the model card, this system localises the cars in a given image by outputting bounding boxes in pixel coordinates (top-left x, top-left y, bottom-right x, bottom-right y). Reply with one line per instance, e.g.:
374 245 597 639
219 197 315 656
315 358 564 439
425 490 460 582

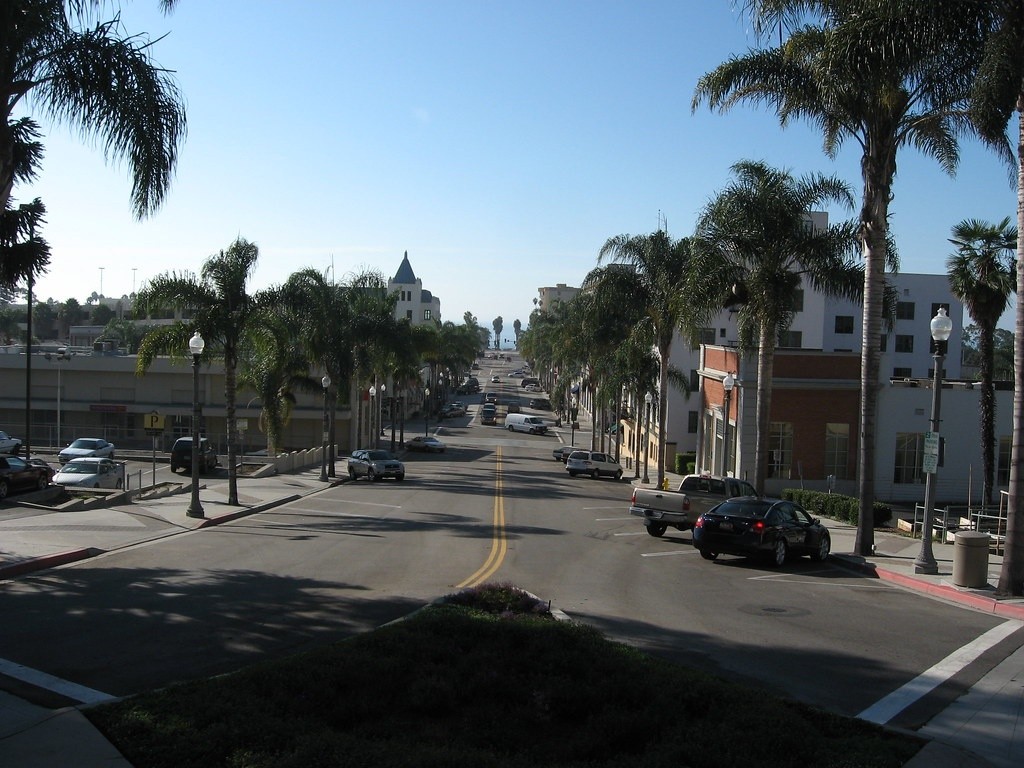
404 437 446 454
552 447 589 464
692 495 833 569
565 450 624 480
440 353 551 424
0 431 124 500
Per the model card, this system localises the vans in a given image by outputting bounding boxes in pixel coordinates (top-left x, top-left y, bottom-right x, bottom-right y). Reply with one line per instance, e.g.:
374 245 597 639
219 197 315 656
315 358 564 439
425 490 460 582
506 413 548 435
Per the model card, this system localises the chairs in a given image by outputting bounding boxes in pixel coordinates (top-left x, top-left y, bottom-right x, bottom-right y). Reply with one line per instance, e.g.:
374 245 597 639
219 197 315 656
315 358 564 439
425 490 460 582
700 483 709 490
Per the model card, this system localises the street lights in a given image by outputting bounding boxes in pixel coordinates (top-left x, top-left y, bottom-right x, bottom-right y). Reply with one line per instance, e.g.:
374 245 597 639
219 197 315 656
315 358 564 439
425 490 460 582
369 386 376 448
641 392 653 484
189 331 205 516
916 308 951 574
720 374 735 478
321 375 331 482
425 389 431 434
381 384 386 436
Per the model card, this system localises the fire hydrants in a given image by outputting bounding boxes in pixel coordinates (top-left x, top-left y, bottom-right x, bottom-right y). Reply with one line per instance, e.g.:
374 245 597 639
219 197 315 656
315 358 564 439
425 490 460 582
661 478 671 490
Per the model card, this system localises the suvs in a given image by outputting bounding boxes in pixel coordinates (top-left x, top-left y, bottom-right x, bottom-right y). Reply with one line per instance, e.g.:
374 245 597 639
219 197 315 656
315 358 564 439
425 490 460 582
171 437 218 475
347 450 404 482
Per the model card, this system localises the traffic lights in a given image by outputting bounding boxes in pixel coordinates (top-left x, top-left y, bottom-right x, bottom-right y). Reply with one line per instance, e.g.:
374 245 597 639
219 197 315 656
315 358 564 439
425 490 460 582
560 395 565 404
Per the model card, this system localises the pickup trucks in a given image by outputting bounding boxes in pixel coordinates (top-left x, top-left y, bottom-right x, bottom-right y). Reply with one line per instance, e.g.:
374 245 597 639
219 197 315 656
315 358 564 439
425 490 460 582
629 475 758 538
